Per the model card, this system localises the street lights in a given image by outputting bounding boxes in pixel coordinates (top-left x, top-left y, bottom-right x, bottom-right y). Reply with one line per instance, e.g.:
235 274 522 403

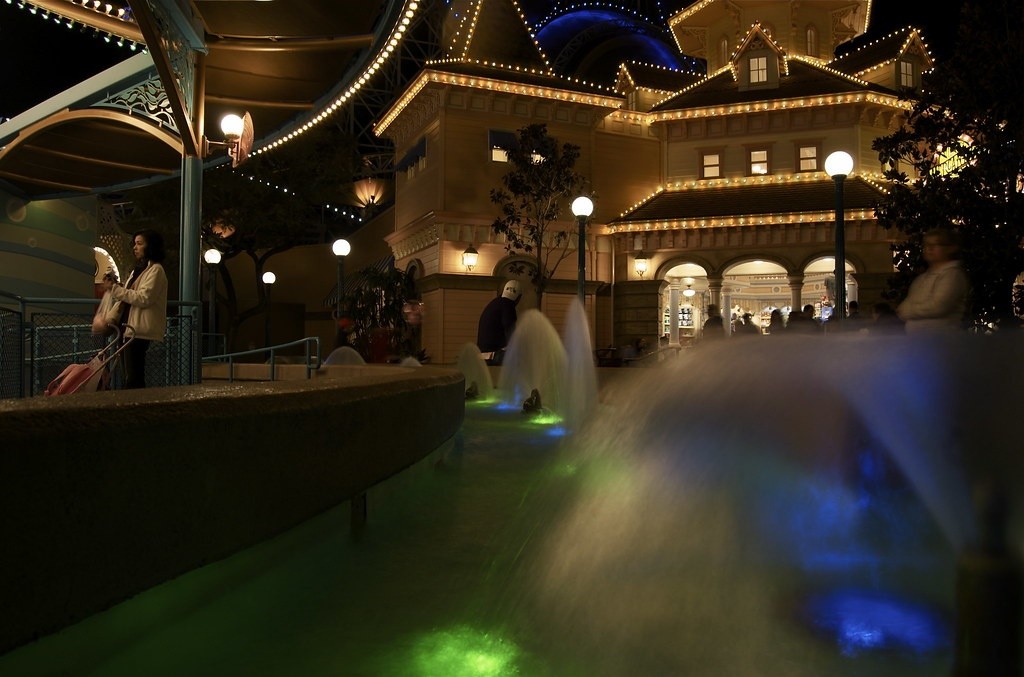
331 238 351 349
825 149 853 320
260 270 276 363
203 248 221 363
570 194 595 317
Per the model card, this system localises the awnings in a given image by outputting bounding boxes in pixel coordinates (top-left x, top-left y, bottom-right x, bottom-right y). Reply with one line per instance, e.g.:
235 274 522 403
322 253 394 307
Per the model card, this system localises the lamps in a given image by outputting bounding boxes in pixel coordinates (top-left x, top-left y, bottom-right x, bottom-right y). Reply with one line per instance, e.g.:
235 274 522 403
201 111 256 169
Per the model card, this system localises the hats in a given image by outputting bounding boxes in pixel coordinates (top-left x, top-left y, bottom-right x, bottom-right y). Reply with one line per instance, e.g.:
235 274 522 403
502 280 522 301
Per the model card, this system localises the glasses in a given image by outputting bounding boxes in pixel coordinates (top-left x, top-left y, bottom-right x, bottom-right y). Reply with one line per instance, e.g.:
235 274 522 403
924 241 947 247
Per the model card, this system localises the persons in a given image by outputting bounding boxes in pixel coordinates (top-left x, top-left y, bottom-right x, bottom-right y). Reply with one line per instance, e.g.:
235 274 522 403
101 229 168 390
895 227 967 333
702 303 906 341
463 280 522 395
335 318 358 350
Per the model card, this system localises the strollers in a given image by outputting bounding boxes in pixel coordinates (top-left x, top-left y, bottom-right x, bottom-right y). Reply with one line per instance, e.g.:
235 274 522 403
41 322 136 395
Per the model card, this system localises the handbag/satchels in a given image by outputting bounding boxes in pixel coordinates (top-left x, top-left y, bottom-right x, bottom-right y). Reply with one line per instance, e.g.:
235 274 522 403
92 286 123 335
